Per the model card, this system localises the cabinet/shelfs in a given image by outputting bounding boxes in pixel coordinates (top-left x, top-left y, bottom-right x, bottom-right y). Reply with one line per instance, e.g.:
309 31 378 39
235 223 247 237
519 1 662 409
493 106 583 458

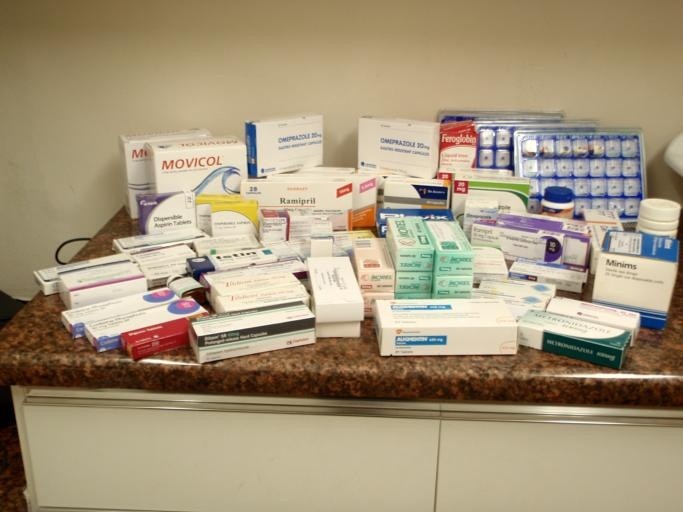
1 200 683 507
